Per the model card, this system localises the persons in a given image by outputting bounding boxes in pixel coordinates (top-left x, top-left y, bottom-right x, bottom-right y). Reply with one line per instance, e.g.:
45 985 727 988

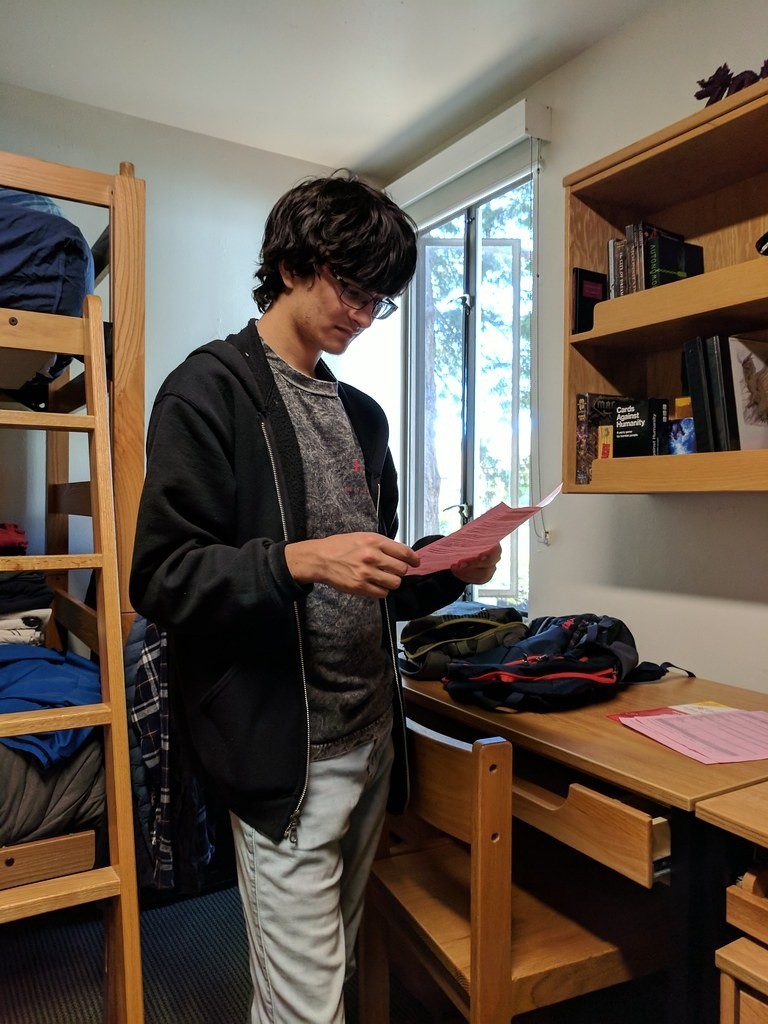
126 166 504 1024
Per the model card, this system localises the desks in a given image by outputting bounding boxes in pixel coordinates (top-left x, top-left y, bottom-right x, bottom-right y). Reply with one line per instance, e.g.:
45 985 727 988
399 672 768 1024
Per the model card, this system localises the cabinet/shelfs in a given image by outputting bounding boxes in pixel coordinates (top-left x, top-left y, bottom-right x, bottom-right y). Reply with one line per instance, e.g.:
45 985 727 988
562 75 768 493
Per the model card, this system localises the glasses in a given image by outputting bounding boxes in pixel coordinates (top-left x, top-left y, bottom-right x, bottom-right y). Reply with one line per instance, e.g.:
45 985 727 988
320 261 398 319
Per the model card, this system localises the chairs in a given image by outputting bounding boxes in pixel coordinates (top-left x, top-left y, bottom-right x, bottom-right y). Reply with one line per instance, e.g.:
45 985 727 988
373 716 689 1024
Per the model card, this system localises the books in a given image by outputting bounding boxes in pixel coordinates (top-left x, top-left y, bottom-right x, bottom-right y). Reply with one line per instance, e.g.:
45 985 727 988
566 314 768 488
565 221 704 338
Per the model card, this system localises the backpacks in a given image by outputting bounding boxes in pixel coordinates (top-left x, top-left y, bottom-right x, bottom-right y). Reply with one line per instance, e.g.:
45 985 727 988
447 605 669 712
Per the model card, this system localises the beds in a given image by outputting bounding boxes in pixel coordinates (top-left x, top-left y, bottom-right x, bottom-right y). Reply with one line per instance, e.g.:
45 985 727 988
0 151 147 1024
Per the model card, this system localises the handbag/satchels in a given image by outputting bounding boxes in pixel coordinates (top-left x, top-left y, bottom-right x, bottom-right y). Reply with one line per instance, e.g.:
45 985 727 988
396 602 532 683
